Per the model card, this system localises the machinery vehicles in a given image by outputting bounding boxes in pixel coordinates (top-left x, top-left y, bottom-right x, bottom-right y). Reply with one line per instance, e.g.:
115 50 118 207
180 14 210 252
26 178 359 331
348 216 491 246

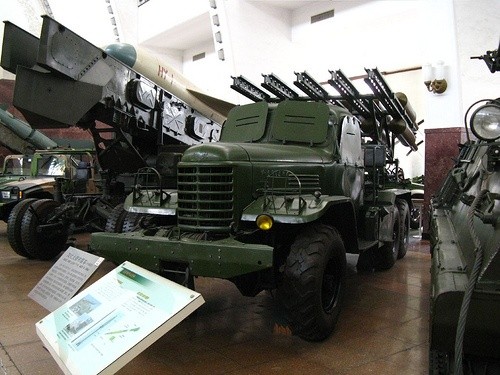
0 14 499 375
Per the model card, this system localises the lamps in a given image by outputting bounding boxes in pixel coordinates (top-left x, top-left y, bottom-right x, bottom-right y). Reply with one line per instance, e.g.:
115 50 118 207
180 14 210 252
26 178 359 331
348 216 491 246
422 59 448 94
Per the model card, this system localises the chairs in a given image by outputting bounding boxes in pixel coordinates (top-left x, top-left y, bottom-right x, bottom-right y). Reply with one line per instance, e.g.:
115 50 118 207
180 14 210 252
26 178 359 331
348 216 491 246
75 162 89 180
